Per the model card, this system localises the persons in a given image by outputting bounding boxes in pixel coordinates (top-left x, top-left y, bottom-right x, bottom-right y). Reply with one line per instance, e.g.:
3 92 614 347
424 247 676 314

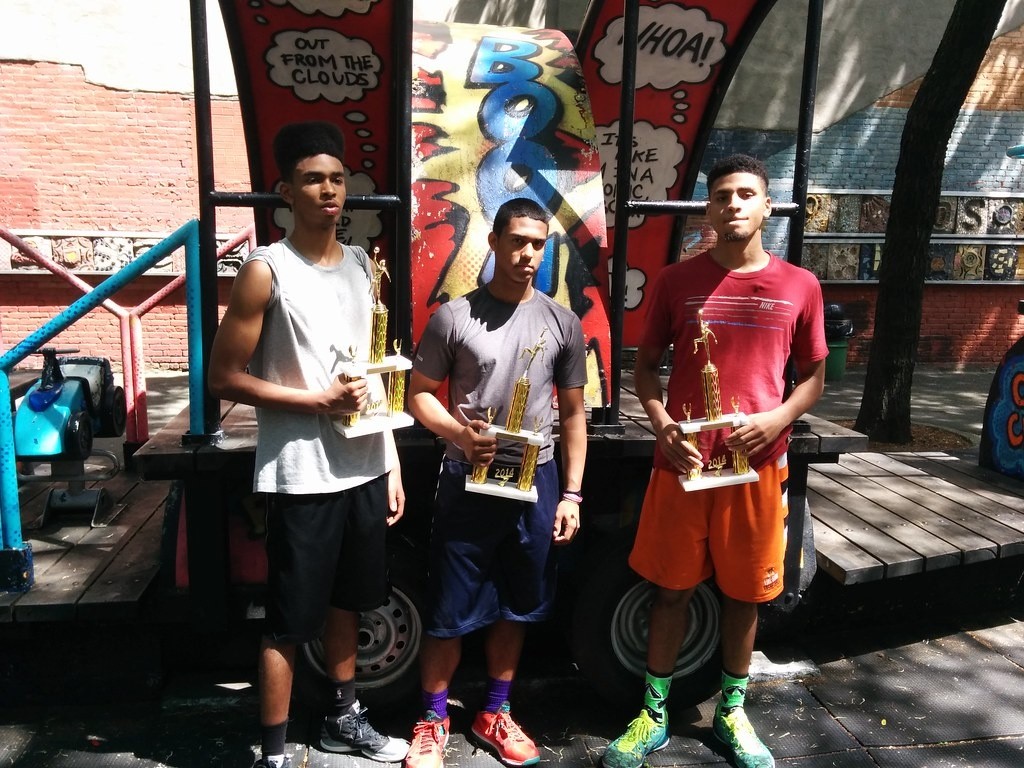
597 143 831 768
397 197 590 766
202 121 414 766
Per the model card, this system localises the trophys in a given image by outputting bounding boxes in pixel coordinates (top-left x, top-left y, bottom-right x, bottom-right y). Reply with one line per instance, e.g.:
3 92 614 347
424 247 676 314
332 241 414 440
675 303 763 496
460 319 550 506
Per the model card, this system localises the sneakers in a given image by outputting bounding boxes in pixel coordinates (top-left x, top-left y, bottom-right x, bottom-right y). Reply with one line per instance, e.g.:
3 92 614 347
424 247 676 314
602 709 669 768
251 753 292 768
322 699 411 763
405 709 450 768
471 701 540 766
712 703 776 768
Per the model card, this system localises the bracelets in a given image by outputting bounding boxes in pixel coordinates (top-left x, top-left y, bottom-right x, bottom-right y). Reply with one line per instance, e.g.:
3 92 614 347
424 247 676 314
562 488 583 504
